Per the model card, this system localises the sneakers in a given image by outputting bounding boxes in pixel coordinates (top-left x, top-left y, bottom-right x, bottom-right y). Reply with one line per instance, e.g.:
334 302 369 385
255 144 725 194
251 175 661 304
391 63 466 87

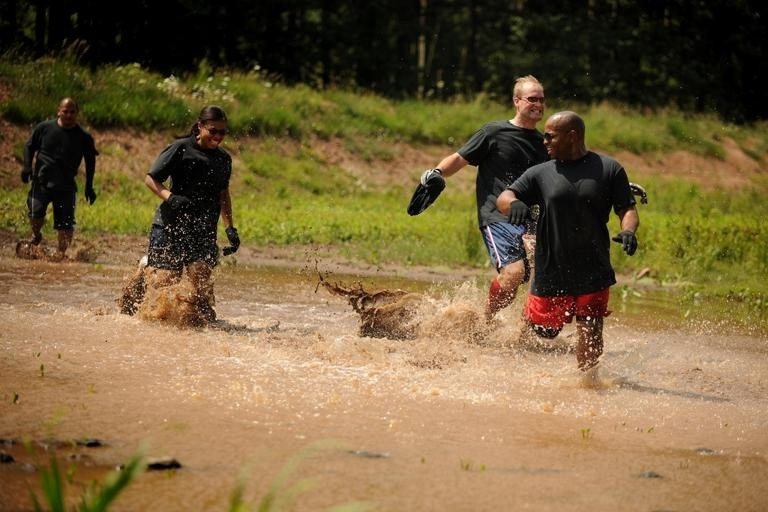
28 232 42 246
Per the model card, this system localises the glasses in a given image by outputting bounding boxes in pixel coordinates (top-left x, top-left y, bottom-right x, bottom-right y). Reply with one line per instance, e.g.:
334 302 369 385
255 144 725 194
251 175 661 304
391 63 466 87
542 133 558 143
520 97 545 103
204 127 226 135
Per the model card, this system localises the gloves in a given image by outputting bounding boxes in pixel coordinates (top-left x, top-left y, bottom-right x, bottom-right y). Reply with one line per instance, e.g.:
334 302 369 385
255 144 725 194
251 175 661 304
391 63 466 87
168 195 195 210
223 228 240 256
85 189 96 204
612 231 637 256
21 167 33 183
421 168 442 188
629 183 647 204
507 199 529 225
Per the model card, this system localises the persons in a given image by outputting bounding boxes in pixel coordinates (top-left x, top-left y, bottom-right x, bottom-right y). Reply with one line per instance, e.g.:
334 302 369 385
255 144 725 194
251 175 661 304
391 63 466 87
20 97 99 256
420 74 648 321
115 105 241 319
495 112 640 370
